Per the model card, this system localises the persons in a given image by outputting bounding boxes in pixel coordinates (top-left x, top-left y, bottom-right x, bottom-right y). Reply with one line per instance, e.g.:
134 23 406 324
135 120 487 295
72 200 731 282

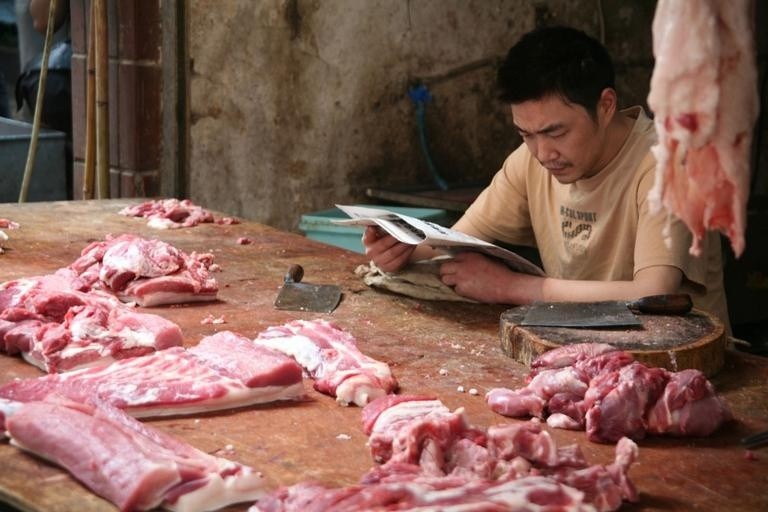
361 20 737 352
29 0 67 34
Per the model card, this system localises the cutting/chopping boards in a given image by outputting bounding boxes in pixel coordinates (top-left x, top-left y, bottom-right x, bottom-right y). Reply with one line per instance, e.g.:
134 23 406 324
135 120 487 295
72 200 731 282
499 301 726 380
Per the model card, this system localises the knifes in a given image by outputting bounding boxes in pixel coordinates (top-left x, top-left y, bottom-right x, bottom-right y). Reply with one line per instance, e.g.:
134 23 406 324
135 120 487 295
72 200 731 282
273 264 341 314
520 293 692 327
